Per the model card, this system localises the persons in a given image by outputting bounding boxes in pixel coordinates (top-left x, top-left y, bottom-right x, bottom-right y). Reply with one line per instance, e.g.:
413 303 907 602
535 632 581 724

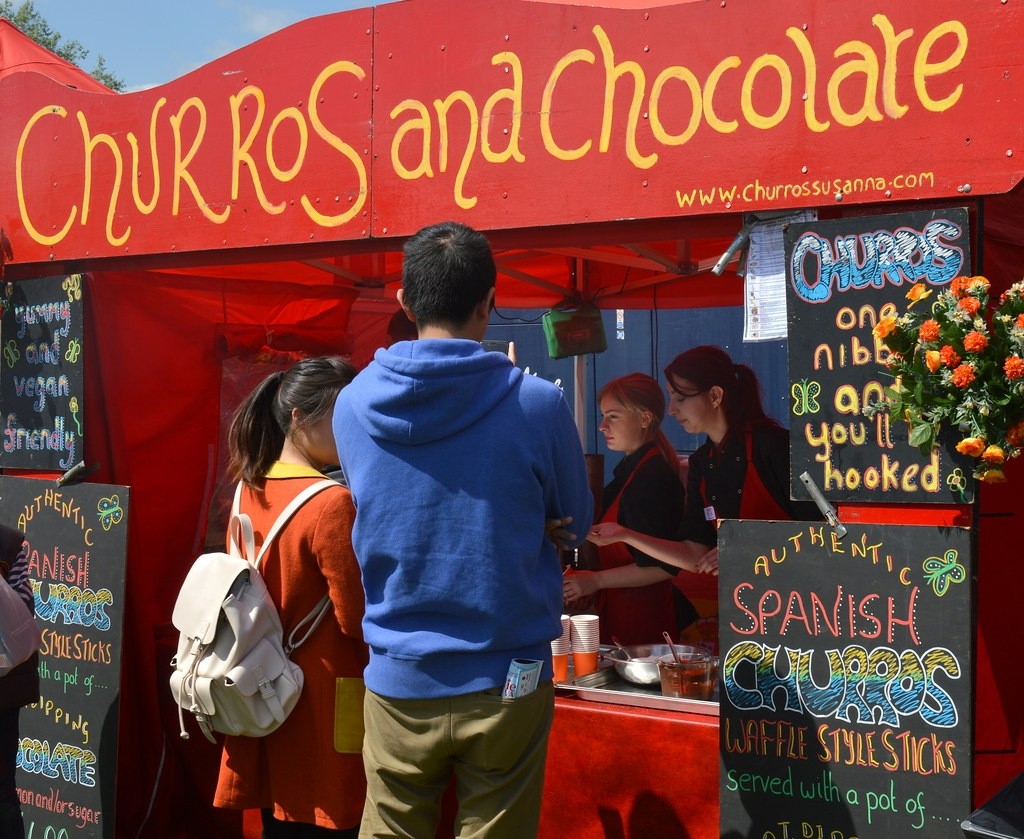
558 372 684 648
211 356 371 839
0 523 44 838
330 221 594 839
589 344 819 659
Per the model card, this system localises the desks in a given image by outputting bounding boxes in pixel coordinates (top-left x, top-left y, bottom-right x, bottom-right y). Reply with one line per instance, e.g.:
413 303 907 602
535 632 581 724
534 692 722 839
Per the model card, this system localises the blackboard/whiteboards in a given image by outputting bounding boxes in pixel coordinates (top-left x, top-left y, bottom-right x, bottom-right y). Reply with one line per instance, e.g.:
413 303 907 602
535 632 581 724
0 480 131 839
711 514 978 832
0 274 90 470
780 200 978 503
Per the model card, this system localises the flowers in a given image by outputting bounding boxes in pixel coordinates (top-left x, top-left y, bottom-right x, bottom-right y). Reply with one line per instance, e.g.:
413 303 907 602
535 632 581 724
860 273 1024 482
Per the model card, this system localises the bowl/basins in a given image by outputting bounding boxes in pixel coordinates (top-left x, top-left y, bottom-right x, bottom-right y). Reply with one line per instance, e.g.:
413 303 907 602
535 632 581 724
603 645 712 684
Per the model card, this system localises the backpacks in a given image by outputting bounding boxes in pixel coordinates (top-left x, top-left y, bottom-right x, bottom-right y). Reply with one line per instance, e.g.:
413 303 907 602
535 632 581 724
168 478 349 743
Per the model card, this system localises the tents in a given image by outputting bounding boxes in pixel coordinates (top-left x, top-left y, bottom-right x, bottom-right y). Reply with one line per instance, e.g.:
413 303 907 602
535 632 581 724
0 0 1024 838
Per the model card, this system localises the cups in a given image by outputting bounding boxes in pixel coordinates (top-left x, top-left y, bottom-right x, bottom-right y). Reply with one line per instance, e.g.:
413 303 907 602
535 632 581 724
550 615 570 684
655 652 719 703
570 615 600 676
561 564 576 581
